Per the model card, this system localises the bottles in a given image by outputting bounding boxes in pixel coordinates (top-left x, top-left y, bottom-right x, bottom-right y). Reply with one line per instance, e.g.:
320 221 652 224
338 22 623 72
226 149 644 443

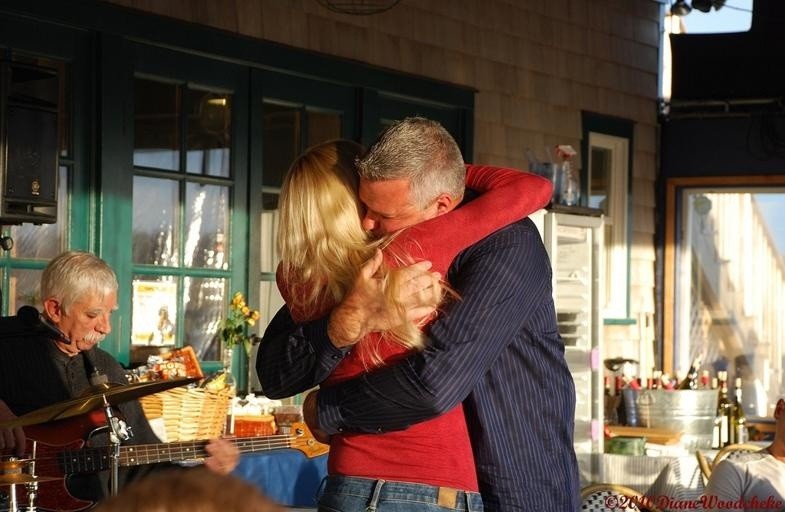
603 356 749 451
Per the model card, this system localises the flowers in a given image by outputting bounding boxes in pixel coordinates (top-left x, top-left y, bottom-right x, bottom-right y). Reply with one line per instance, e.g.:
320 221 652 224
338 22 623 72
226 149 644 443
218 290 259 352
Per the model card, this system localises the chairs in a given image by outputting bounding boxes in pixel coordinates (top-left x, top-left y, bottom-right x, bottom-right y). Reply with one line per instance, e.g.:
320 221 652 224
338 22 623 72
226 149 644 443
580 443 785 512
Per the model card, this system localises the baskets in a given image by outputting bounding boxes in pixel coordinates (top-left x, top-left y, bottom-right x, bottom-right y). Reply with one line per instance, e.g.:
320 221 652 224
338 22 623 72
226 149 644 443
139 386 236 442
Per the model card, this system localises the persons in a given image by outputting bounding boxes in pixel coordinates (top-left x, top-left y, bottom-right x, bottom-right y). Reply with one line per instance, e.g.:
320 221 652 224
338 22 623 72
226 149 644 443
705 397 785 512
0 249 240 497
256 117 582 510
275 136 553 510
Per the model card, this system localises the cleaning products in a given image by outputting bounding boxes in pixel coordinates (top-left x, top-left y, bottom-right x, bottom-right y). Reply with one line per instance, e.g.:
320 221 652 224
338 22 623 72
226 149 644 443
557 142 582 206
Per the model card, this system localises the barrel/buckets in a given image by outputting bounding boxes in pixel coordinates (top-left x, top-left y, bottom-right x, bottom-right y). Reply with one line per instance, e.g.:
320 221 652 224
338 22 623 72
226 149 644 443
619 386 721 455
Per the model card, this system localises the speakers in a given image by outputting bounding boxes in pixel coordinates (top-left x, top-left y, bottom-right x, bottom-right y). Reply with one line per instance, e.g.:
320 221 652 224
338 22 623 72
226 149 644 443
0 60 61 227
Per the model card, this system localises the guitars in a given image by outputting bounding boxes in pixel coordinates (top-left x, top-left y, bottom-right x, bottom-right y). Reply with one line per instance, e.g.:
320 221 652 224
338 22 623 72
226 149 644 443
1 407 329 510
